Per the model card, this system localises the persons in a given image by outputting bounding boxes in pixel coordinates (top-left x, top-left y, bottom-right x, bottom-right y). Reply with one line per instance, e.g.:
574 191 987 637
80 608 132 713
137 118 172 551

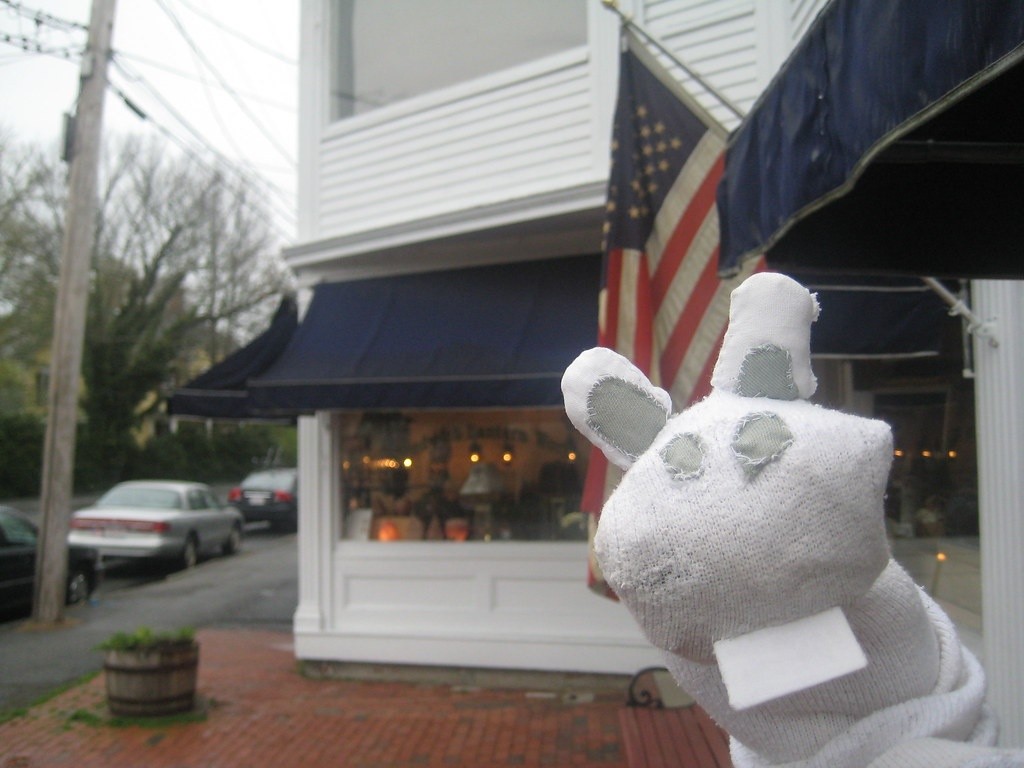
344 437 583 539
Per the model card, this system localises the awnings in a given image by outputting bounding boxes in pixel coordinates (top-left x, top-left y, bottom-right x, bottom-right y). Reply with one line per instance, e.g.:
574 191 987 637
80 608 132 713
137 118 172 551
713 0 1023 277
242 249 598 416
165 292 302 426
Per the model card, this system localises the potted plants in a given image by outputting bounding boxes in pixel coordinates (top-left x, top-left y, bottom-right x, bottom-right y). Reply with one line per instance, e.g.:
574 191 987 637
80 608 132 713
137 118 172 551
94 623 201 720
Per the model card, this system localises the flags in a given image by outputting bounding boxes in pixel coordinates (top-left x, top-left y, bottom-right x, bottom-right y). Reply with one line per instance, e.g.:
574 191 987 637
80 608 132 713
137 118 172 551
583 17 772 603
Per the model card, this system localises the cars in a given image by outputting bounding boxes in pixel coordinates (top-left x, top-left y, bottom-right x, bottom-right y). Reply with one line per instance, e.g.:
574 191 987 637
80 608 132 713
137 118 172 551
0 503 96 628
229 465 297 535
66 476 246 586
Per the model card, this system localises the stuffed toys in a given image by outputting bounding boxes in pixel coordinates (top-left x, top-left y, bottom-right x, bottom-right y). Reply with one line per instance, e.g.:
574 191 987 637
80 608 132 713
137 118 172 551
557 271 1024 768
